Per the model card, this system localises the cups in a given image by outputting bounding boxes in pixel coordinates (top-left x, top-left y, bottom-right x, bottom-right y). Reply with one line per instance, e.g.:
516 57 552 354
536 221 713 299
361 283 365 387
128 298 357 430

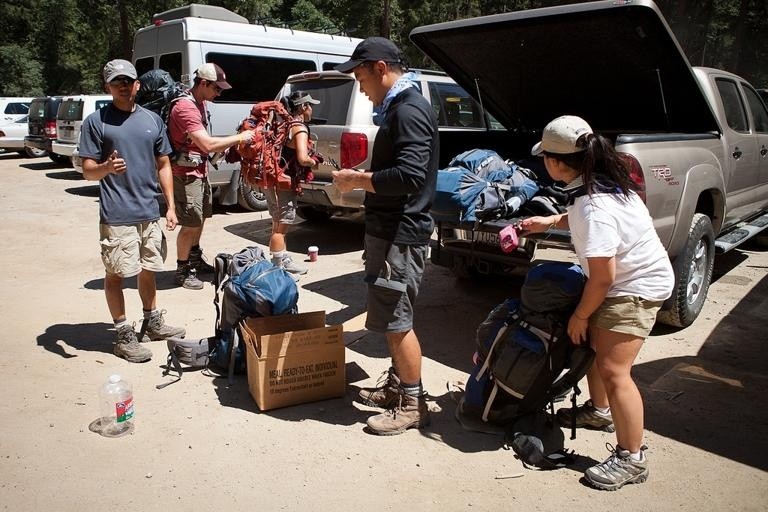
308 246 319 262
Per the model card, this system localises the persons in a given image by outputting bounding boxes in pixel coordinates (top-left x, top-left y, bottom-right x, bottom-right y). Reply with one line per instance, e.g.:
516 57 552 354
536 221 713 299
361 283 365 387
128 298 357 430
167 63 256 290
332 37 438 435
261 91 321 282
77 60 186 364
516 114 675 490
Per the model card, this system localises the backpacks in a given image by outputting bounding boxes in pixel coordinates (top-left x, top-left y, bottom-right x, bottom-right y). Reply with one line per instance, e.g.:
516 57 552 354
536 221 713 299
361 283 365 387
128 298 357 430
135 71 210 164
456 261 599 470
430 147 540 230
234 100 311 231
157 245 299 390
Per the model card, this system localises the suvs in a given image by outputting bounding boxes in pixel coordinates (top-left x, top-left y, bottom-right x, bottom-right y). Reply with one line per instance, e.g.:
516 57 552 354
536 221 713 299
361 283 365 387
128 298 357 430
268 69 505 224
51 93 113 172
0 97 47 157
24 96 69 167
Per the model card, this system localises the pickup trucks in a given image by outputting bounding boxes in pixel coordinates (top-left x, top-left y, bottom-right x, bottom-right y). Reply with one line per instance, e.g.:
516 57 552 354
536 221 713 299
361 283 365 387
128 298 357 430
409 0 764 329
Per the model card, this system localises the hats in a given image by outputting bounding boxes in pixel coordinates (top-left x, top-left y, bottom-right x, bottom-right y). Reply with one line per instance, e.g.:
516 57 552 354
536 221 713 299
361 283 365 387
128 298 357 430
333 35 401 74
196 62 232 89
293 94 320 106
531 114 593 156
103 59 137 84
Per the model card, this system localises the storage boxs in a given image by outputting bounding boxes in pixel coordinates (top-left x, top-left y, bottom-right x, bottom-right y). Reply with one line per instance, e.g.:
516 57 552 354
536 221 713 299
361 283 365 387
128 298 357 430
239 308 345 411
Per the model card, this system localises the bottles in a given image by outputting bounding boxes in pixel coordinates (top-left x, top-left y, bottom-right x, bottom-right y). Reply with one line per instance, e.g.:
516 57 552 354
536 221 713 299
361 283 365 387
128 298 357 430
98 374 134 438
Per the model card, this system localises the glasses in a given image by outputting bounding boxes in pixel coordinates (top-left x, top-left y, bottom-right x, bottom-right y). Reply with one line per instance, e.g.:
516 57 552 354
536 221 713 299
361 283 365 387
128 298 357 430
110 77 134 86
209 82 223 94
328 156 342 171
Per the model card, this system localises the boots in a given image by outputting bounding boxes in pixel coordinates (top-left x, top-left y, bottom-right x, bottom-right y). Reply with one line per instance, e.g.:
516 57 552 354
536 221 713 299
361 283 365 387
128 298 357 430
367 387 431 435
356 367 399 408
139 309 186 342
113 321 152 363
191 251 214 277
175 263 203 289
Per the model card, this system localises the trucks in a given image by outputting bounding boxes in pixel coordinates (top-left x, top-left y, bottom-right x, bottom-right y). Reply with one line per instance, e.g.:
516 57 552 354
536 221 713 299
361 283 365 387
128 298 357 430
132 5 366 210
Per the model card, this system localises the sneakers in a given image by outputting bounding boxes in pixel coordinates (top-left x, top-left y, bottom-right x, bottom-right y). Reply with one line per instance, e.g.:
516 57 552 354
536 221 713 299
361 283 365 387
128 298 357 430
585 444 649 491
280 266 299 282
283 252 308 275
557 399 616 433
